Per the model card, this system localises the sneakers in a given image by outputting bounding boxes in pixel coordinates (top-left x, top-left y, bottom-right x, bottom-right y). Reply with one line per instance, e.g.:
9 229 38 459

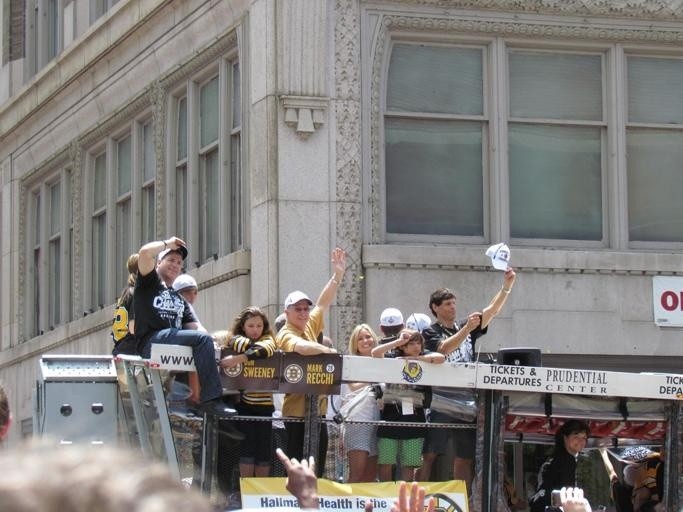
196 399 240 418
216 420 248 442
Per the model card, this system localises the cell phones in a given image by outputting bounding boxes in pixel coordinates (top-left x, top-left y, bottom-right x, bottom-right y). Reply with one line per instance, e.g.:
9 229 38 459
550 491 565 508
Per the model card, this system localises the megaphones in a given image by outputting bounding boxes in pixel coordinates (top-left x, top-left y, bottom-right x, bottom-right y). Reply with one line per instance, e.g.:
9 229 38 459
498 348 541 367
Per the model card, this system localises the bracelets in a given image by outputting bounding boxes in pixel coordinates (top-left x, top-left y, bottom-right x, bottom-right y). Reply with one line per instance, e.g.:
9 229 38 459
161 240 167 252
331 276 340 286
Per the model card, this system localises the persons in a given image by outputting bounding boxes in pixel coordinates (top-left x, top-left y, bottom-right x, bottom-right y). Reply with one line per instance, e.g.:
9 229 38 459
528 416 667 512
0 432 229 509
271 243 346 477
136 307 276 498
0 386 16 447
131 232 235 417
276 449 435 512
111 252 154 356
421 260 516 493
171 273 218 411
326 307 446 482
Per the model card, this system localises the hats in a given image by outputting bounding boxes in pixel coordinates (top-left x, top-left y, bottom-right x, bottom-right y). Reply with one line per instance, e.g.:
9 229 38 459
405 312 432 331
379 307 403 327
170 273 198 292
157 243 189 262
284 290 313 309
484 242 512 272
